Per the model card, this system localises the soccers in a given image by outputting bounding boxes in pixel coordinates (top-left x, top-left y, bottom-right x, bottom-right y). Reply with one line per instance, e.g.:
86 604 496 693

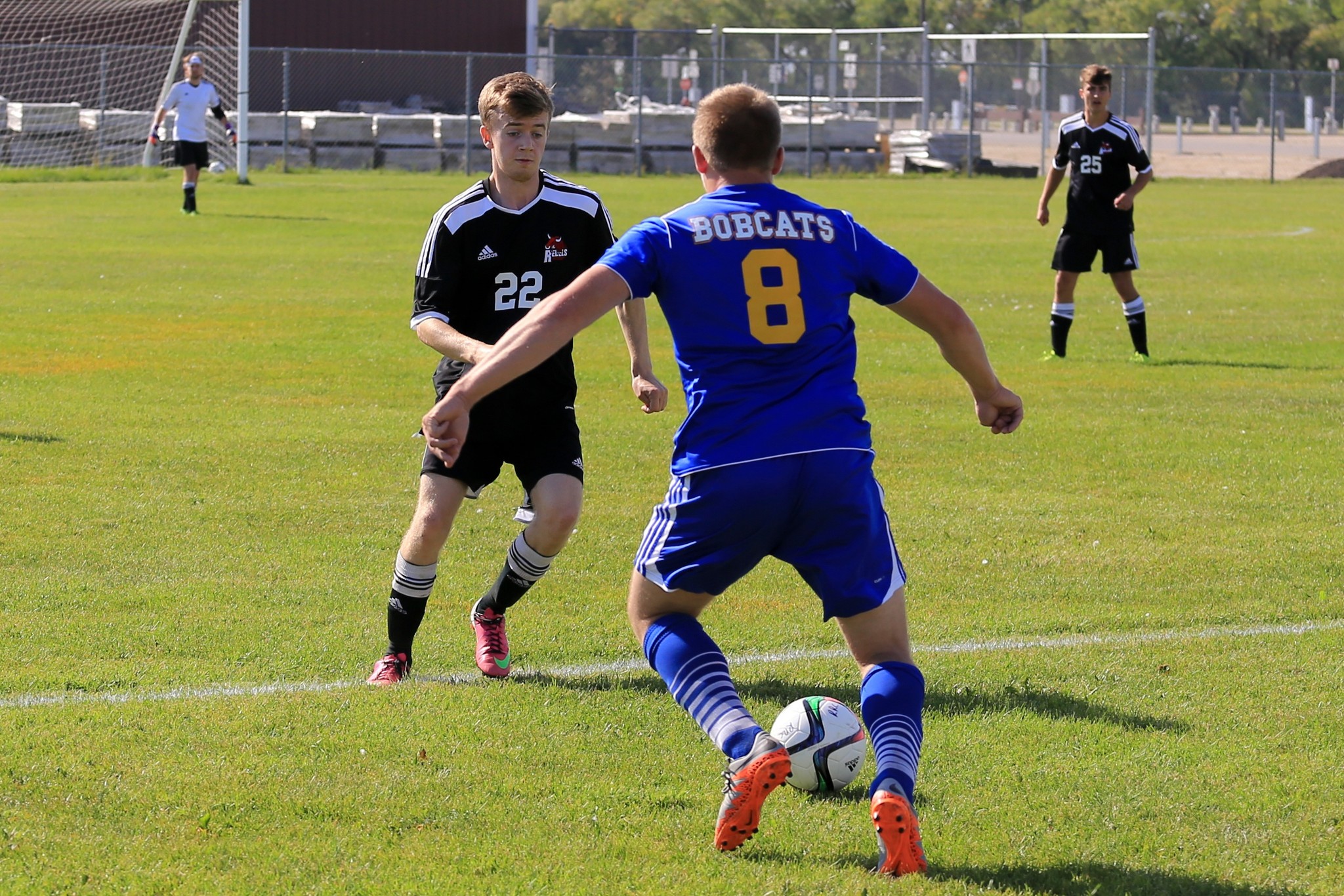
769 695 868 796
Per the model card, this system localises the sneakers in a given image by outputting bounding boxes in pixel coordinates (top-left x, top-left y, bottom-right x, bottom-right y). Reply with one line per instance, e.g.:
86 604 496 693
1038 348 1065 362
870 789 929 876
1133 351 1154 366
470 596 511 678
713 732 793 853
364 653 411 686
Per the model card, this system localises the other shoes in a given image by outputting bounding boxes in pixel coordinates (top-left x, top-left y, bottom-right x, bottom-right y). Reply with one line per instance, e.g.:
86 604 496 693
181 207 200 216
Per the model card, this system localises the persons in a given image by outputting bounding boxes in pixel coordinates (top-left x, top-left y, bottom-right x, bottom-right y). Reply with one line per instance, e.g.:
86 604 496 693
148 52 238 215
421 80 1025 880
1037 64 1155 363
364 70 670 683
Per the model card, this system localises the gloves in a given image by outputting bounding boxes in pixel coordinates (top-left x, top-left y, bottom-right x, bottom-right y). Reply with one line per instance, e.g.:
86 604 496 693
148 124 161 146
225 124 237 148
